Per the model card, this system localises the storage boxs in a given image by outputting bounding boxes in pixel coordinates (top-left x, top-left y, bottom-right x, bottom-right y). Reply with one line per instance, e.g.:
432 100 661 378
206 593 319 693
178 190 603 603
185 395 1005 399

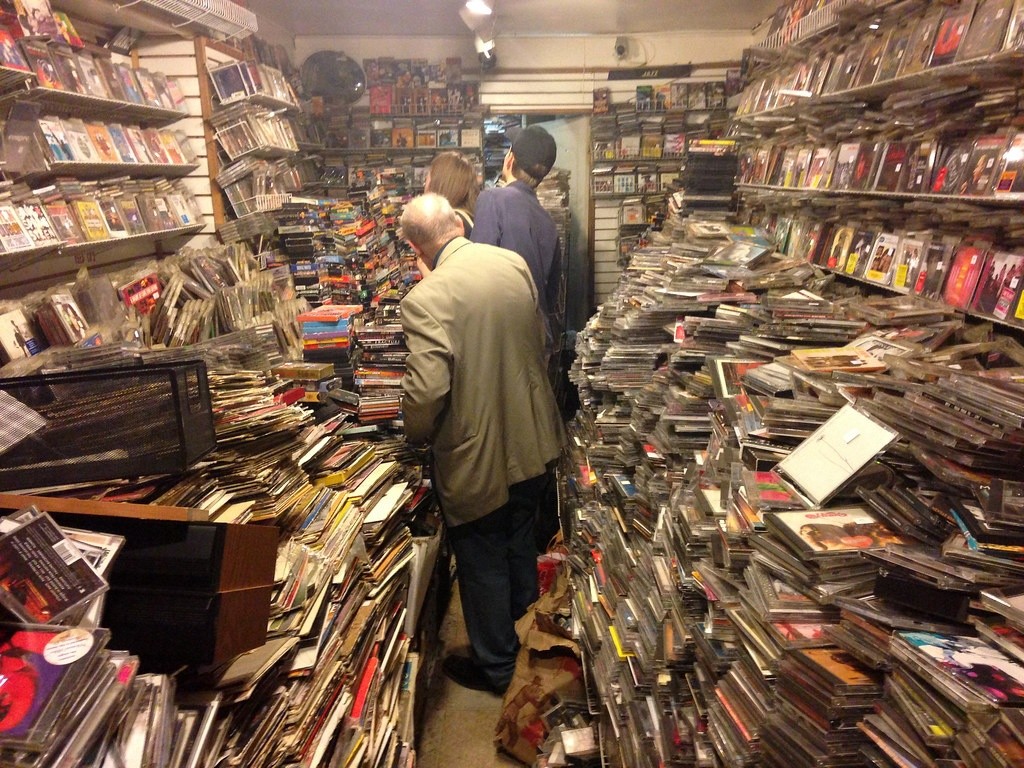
0 495 280 666
0 359 218 491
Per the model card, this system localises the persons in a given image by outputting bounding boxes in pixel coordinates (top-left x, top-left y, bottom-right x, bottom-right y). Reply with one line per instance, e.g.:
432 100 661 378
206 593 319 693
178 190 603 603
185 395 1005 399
398 190 569 697
424 124 564 557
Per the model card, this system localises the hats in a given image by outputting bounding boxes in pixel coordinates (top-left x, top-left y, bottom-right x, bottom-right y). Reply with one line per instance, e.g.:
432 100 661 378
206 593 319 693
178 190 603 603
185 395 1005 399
504 127 557 180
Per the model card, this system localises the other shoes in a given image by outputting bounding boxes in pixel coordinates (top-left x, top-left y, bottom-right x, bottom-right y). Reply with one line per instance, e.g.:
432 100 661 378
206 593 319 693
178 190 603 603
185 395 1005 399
534 520 547 552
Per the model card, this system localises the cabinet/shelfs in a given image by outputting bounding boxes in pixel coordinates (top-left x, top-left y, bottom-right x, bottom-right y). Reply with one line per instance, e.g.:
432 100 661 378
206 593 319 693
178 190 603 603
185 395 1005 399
0 0 491 273
588 0 1024 330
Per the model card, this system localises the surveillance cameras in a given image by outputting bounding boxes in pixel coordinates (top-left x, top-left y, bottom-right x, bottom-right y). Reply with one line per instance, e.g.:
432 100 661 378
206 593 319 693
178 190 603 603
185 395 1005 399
613 37 629 59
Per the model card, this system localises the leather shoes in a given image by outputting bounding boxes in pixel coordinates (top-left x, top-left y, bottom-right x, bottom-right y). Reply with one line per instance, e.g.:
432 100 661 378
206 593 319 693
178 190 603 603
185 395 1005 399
442 653 507 695
